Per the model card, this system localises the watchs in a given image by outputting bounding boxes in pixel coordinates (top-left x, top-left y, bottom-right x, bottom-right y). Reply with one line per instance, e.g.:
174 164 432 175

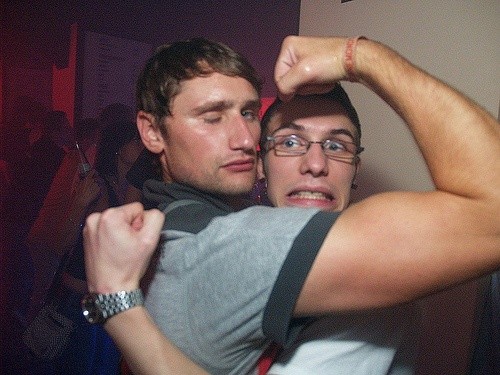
81 289 146 327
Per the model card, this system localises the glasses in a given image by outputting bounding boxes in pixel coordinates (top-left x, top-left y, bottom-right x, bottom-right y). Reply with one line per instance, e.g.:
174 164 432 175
260 133 365 160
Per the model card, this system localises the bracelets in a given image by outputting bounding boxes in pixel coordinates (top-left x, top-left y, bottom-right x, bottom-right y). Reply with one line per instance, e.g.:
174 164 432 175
342 34 368 83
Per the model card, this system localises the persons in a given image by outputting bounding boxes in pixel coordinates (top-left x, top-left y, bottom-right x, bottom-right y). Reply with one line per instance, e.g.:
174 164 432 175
78 35 500 375
82 80 424 375
17 102 162 374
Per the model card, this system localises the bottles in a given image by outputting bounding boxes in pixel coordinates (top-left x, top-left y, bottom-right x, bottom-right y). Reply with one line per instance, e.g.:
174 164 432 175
69 135 97 178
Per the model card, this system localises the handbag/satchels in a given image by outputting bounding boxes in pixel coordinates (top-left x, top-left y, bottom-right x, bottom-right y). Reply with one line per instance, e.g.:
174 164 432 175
22 308 77 366
